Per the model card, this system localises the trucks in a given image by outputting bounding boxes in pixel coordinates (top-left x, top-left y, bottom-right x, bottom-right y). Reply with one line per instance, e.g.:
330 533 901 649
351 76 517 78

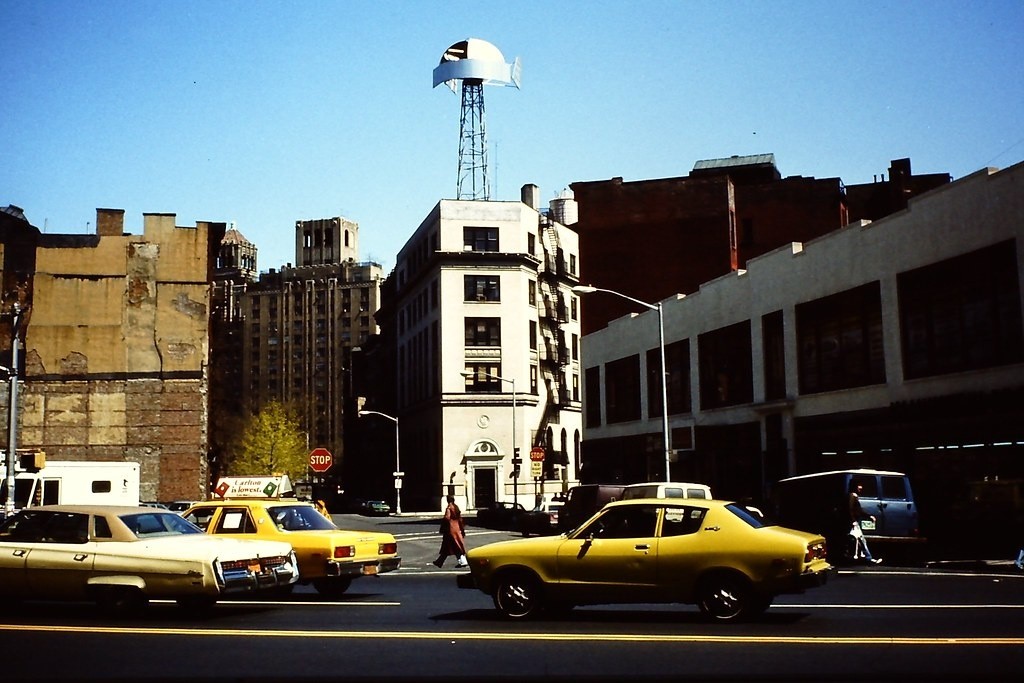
0 461 140 525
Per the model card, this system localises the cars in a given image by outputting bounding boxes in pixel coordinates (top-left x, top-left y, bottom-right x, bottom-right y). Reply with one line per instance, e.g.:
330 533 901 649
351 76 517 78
141 476 400 601
480 502 565 530
0 505 300 620
466 499 830 625
360 501 390 516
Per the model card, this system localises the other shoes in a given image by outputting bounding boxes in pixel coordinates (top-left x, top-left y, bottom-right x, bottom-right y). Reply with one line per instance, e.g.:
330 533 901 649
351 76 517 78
868 558 882 567
433 561 441 568
455 564 469 568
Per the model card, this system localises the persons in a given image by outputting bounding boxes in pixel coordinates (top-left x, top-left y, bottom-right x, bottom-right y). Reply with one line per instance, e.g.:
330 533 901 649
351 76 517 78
847 484 882 564
433 495 468 568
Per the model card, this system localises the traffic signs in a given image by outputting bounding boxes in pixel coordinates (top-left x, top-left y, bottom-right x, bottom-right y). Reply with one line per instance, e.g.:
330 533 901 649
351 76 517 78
393 472 404 477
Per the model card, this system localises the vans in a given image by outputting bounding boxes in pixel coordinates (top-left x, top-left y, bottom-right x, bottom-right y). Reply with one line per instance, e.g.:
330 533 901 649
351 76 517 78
562 483 763 536
779 470 922 568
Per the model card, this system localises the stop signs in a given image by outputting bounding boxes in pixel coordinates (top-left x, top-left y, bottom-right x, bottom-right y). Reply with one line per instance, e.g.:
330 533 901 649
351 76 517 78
309 448 332 472
530 448 545 462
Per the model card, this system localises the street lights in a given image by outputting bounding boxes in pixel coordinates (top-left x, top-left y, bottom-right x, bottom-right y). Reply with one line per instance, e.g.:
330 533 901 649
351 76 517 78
358 411 401 514
460 371 518 509
570 287 669 483
